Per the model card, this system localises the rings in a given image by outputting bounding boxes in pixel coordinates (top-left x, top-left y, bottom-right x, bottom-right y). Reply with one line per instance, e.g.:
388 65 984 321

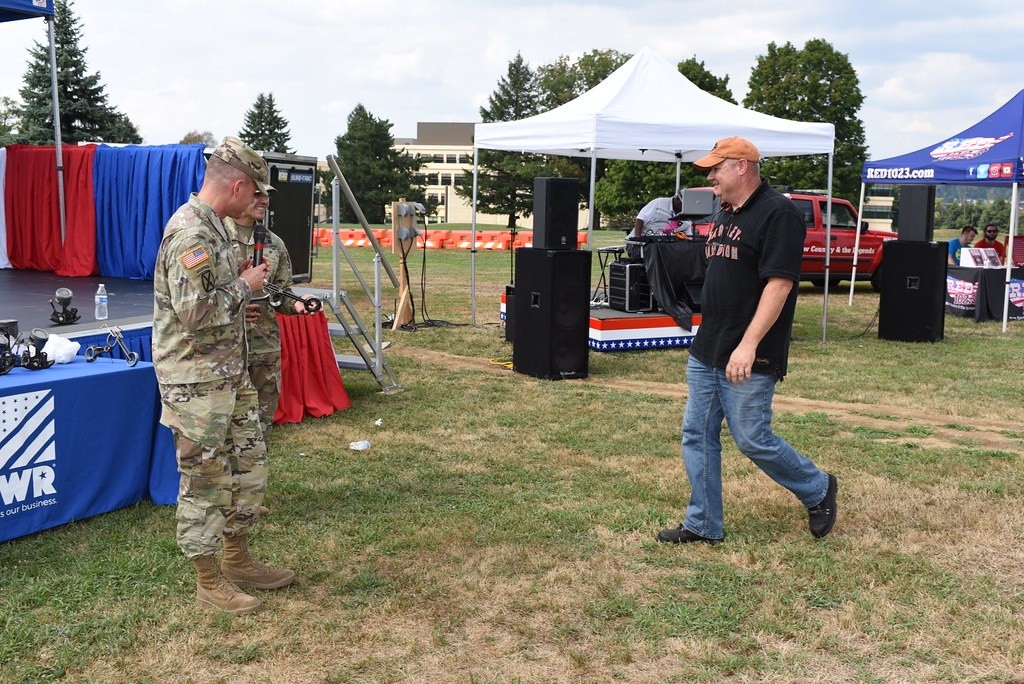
738 369 744 373
263 279 269 285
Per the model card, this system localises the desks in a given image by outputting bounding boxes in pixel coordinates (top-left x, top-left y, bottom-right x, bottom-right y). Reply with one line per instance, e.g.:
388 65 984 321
940 267 1024 322
0 356 180 543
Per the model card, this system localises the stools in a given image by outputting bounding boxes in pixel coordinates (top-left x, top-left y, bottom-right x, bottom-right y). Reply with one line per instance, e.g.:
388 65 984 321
592 249 624 305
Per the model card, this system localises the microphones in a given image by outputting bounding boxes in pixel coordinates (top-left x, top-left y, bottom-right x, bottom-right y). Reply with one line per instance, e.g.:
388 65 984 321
252 225 266 267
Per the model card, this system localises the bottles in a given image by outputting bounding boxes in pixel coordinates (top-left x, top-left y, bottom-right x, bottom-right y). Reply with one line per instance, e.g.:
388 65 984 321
94 284 108 320
349 440 370 450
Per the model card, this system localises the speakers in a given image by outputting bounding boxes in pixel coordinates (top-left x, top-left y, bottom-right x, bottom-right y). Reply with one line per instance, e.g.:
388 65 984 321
512 247 592 380
533 176 580 250
877 240 949 344
608 262 652 312
897 185 936 242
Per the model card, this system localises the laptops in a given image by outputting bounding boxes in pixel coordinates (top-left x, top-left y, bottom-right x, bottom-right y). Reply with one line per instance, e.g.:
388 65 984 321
669 188 714 220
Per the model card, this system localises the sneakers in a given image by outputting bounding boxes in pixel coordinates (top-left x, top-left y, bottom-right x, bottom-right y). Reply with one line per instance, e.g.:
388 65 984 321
659 523 725 544
808 473 837 538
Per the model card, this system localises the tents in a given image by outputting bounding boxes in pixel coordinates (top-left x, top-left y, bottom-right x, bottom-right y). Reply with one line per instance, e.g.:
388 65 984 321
0 0 68 247
848 86 1024 335
470 46 836 345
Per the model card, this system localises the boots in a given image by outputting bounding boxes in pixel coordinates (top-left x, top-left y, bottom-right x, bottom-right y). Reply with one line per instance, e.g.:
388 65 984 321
193 554 260 616
222 534 295 589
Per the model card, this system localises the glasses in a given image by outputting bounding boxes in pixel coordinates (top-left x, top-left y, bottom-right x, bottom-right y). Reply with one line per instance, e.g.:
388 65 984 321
967 227 976 232
986 230 996 233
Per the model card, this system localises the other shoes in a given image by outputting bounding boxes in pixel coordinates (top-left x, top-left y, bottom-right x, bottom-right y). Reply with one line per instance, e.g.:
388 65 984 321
260 506 268 516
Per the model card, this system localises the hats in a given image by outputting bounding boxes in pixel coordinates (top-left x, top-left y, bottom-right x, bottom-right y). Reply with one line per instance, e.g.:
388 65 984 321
254 177 278 193
213 136 269 196
693 136 760 170
677 188 687 202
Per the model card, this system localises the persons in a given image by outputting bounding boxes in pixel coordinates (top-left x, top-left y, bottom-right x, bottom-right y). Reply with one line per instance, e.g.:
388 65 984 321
151 133 324 616
974 224 1006 266
624 186 688 257
948 225 979 266
657 135 838 545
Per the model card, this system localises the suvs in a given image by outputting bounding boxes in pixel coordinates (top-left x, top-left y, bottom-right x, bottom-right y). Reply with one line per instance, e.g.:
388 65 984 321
691 187 898 292
